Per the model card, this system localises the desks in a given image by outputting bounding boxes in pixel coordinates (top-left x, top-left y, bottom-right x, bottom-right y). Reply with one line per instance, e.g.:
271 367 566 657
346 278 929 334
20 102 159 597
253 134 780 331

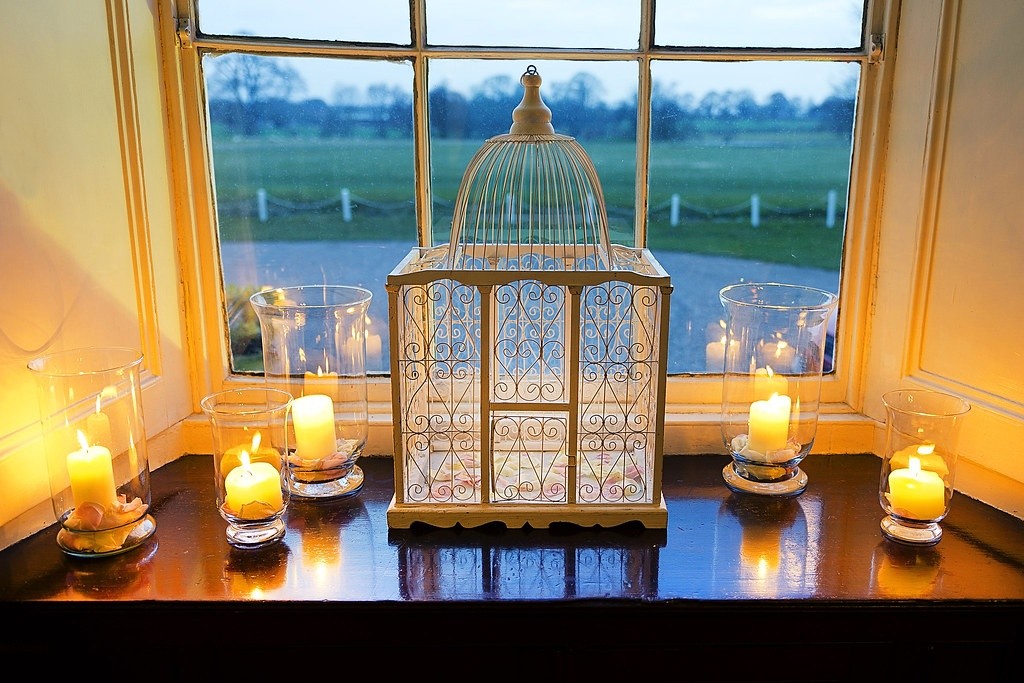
1 457 1024 683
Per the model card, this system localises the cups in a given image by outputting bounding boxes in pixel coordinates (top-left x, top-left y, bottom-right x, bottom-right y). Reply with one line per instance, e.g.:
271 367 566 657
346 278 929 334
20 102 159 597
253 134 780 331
251 285 373 502
879 389 971 548
200 387 294 550
718 282 838 497
27 347 156 557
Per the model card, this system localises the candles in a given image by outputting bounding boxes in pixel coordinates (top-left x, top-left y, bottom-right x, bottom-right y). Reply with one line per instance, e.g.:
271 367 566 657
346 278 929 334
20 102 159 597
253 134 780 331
88 395 112 443
304 365 338 401
291 395 338 460
66 429 118 511
748 392 791 454
705 319 797 375
888 455 946 520
225 449 284 515
